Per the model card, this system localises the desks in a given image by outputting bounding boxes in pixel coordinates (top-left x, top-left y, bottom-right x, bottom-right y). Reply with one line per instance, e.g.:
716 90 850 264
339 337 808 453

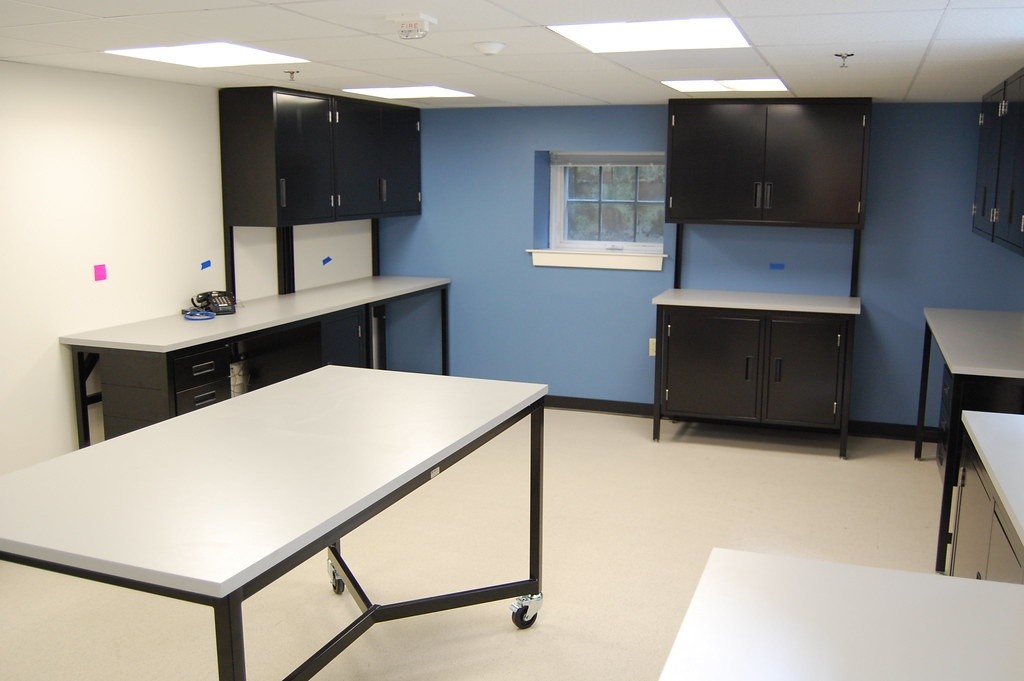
0 363 549 681
59 275 452 450
651 288 861 458
659 548 1024 680
914 306 1024 572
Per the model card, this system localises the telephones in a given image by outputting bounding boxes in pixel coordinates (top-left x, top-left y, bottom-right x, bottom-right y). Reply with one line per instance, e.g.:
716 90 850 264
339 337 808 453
197 290 236 315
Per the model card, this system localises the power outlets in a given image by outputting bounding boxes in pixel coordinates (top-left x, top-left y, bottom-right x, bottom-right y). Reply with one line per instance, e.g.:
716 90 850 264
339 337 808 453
648 337 656 356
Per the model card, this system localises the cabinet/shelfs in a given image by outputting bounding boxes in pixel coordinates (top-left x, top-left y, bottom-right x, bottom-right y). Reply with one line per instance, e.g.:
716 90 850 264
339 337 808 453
935 365 1024 486
950 409 1024 584
664 97 873 228
98 346 232 441
247 308 367 392
973 67 1024 253
219 84 333 226
333 94 422 222
662 308 847 430
